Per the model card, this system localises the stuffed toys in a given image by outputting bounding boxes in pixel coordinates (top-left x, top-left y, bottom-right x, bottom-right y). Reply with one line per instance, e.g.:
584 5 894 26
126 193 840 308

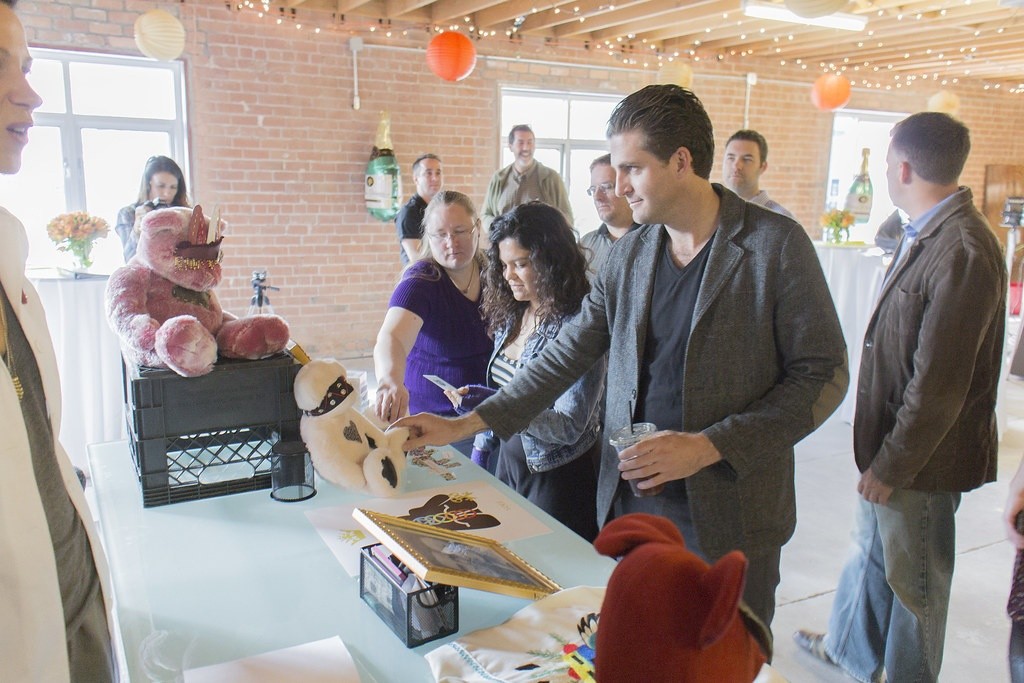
294 359 408 498
106 206 289 376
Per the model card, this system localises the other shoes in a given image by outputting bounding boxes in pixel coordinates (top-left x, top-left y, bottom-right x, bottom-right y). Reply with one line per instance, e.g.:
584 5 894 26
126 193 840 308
793 630 881 683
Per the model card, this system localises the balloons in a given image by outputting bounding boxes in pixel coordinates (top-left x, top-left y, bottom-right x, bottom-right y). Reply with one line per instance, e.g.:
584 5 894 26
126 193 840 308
425 31 476 81
132 9 186 63
928 91 961 118
811 72 852 111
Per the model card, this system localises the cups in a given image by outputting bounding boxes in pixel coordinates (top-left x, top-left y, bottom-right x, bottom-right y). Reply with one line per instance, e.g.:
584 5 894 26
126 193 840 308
610 423 664 498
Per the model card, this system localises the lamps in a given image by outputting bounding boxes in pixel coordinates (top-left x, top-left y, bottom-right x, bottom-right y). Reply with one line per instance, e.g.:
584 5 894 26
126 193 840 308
743 2 868 30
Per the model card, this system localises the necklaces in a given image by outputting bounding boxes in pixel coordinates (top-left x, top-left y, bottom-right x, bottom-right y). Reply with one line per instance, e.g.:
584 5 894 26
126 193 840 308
449 263 475 293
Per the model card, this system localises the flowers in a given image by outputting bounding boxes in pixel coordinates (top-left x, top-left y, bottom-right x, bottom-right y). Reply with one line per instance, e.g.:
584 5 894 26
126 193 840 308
820 210 855 243
45 211 109 241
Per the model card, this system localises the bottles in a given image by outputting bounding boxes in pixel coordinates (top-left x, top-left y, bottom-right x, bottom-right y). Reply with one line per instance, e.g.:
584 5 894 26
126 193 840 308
365 110 403 221
842 149 874 223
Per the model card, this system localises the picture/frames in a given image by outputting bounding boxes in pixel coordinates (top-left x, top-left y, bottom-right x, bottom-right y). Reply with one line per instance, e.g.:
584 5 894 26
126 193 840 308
352 507 565 598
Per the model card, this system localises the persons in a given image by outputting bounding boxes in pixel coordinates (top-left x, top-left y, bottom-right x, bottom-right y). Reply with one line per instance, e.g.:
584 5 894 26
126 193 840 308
373 191 493 460
578 152 641 290
0 0 127 683
386 84 851 631
1004 453 1024 683
481 126 574 231
393 154 440 267
115 156 186 264
723 130 794 218
797 112 1009 683
442 202 607 523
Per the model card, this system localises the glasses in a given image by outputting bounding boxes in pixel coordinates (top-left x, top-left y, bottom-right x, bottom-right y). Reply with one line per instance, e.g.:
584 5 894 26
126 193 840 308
428 221 476 242
587 182 615 196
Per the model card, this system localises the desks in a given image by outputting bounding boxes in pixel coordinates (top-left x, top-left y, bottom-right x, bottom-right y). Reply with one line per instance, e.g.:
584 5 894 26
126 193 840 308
810 241 884 352
85 410 818 683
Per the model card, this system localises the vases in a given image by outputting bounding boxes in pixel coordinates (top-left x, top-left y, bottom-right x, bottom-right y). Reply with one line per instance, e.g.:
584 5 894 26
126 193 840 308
68 245 93 269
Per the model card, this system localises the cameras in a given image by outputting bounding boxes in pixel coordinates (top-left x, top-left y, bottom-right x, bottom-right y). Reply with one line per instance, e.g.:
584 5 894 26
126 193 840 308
153 198 169 209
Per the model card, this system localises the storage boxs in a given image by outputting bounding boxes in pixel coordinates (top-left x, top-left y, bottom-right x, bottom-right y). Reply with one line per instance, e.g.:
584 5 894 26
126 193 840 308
357 545 459 651
120 343 305 510
